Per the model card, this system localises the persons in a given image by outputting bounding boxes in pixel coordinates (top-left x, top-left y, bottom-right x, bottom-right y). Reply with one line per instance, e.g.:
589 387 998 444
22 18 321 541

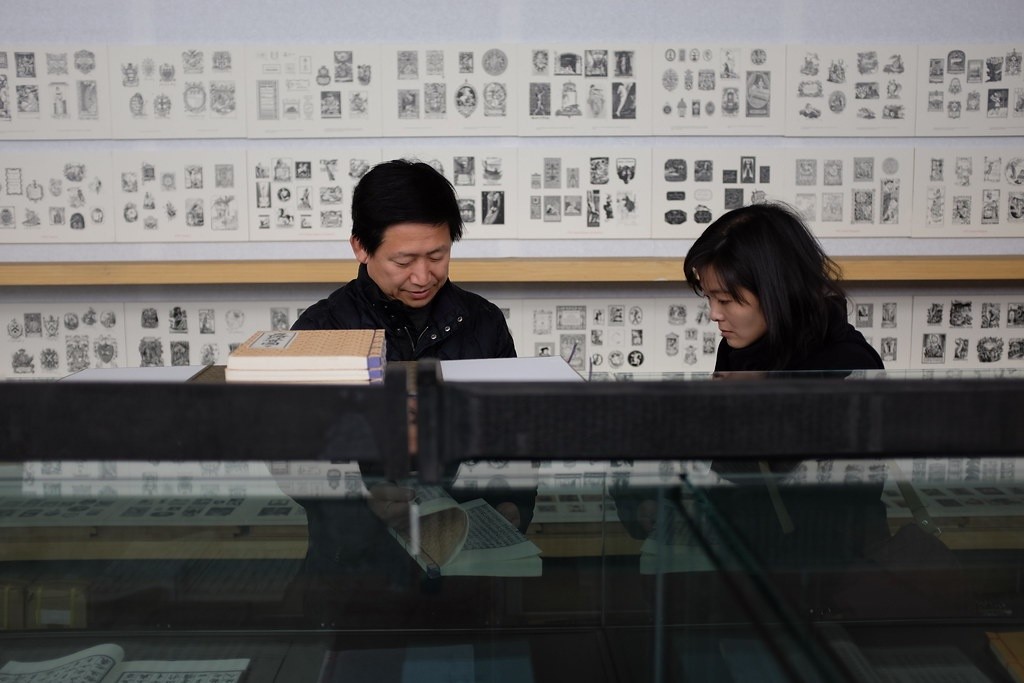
285 154 536 629
637 202 891 576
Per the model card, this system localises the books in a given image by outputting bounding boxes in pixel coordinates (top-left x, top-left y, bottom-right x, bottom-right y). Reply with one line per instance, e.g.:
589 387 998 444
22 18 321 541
385 474 547 582
315 644 475 682
714 632 990 683
986 627 1023 683
0 643 250 683
223 329 388 387
